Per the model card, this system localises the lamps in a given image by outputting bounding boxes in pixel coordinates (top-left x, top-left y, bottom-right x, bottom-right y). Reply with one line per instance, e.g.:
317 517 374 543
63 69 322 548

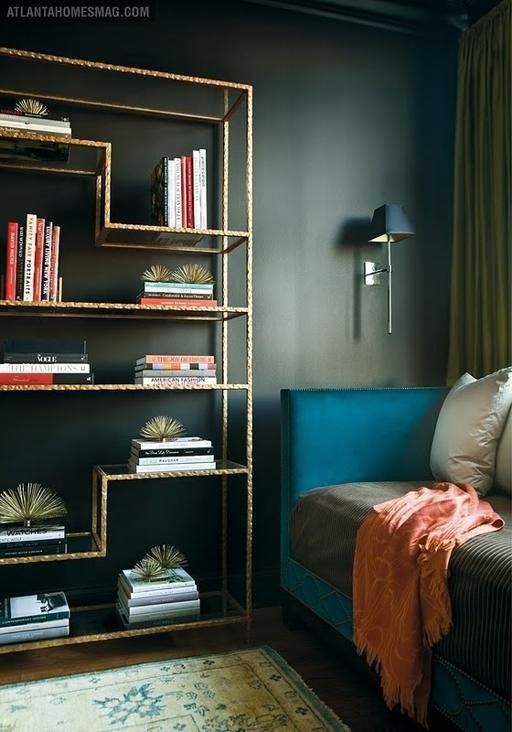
364 200 417 335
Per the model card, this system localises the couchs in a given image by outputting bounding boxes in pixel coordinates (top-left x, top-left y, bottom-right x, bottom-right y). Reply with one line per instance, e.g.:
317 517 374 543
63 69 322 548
271 384 512 732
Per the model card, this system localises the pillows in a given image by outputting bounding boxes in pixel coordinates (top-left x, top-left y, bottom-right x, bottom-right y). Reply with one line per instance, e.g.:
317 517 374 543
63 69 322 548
492 403 512 493
429 364 512 497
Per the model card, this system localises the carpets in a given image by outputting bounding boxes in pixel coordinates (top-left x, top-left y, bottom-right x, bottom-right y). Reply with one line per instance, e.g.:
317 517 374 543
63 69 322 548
0 643 353 732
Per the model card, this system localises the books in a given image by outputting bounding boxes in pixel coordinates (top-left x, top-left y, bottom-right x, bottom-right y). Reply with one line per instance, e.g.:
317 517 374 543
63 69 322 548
0 111 226 642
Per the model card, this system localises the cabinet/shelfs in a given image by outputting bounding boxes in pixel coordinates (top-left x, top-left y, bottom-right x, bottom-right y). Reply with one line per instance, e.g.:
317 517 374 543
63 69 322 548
0 48 256 644
